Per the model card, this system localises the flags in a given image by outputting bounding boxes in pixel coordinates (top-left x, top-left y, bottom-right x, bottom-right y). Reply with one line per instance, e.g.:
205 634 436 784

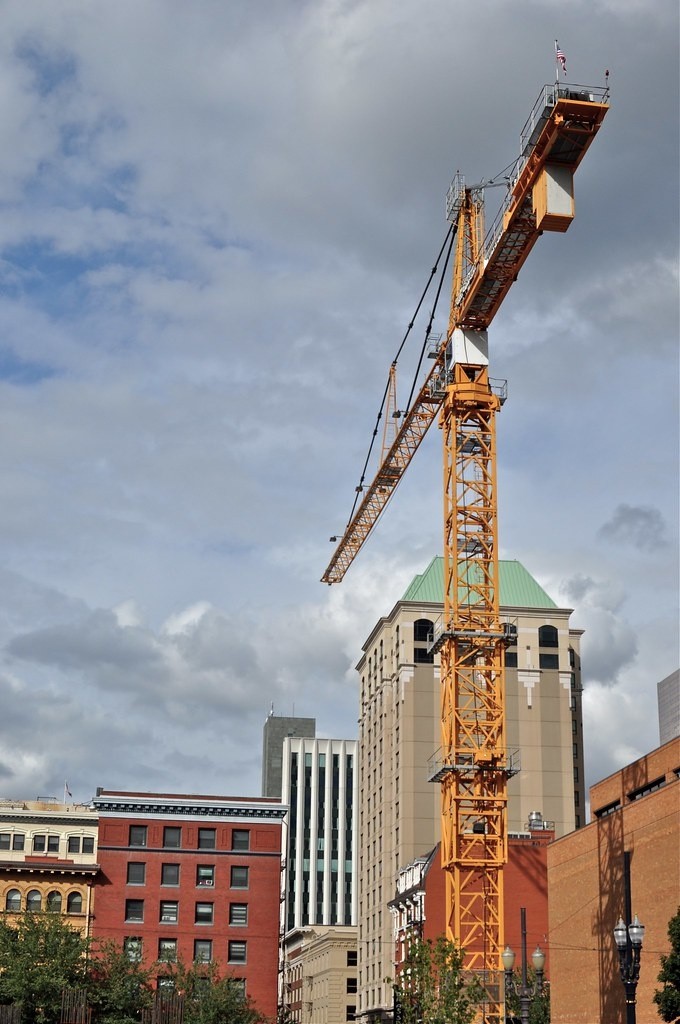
66 782 72 798
556 44 567 77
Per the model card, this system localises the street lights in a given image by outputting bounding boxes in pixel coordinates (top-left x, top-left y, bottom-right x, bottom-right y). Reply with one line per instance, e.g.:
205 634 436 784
500 907 546 1024
616 914 645 1023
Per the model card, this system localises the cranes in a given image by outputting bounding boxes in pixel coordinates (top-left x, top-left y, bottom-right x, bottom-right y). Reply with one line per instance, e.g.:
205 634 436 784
320 28 614 1017
